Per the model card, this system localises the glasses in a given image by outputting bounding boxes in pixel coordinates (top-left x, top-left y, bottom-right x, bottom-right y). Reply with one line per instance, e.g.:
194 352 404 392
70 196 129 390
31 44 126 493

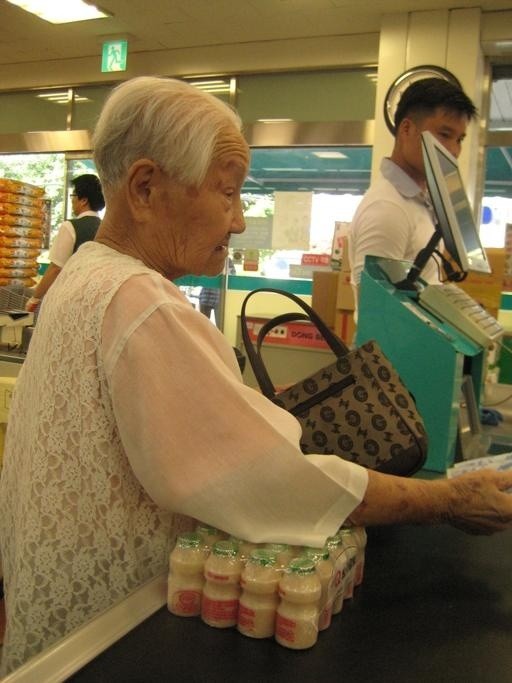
70 194 82 198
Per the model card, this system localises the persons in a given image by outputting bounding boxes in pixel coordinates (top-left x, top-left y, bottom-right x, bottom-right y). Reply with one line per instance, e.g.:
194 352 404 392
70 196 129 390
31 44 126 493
199 256 236 332
0 77 510 679
25 174 105 329
347 77 480 349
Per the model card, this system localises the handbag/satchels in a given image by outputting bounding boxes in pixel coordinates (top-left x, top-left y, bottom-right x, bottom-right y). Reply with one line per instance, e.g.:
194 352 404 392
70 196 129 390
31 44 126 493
266 339 431 480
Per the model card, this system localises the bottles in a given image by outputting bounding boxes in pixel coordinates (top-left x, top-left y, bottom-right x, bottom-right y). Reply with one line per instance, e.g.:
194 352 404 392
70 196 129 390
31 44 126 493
166 520 366 649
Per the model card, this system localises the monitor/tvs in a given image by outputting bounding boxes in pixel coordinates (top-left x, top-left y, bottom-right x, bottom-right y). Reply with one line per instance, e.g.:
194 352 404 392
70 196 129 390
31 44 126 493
419 129 492 274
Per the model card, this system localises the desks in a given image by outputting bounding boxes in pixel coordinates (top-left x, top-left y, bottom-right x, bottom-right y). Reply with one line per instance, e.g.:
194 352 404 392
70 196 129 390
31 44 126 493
1 471 510 679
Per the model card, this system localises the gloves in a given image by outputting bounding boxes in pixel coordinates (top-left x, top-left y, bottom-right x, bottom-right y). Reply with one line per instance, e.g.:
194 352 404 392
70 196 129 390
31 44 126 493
26 296 41 313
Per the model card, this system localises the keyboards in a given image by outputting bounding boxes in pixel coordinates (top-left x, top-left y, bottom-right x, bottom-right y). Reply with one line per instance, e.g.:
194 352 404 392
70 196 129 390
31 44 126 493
419 282 506 348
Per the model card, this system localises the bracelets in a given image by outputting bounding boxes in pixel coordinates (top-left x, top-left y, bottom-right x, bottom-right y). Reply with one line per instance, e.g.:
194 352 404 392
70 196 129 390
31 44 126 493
29 296 42 305
